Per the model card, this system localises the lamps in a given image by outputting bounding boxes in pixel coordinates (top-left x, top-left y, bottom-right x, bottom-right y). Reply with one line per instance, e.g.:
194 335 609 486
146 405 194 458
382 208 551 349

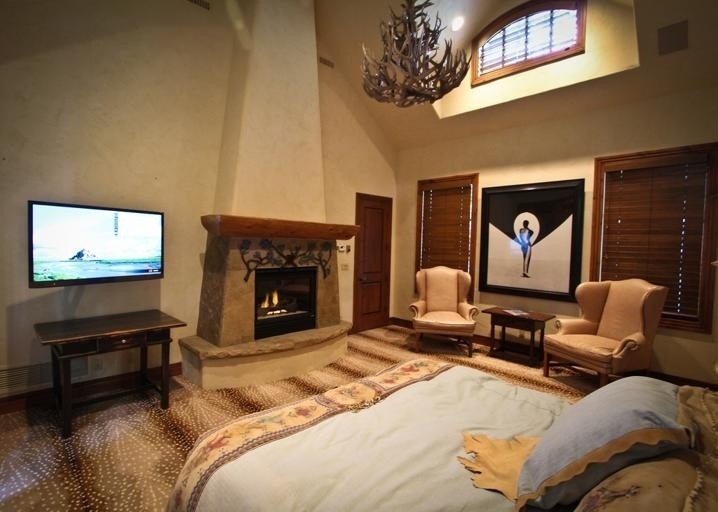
360 1 473 108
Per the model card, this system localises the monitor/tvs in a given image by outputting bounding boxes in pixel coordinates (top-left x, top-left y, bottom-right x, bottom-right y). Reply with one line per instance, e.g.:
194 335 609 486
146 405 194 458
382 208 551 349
27 200 165 289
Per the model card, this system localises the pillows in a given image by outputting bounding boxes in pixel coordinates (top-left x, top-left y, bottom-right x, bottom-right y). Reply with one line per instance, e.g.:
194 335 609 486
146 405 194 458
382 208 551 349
518 373 697 509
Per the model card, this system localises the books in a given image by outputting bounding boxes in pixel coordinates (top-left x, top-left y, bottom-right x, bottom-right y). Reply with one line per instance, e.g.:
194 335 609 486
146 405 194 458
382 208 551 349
503 308 529 317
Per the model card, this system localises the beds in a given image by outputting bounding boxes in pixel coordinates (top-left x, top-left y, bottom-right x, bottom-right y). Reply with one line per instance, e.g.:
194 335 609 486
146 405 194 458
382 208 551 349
167 354 595 512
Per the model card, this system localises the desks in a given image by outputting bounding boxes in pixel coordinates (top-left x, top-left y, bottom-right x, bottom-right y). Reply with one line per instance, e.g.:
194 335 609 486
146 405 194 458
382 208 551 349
33 309 187 440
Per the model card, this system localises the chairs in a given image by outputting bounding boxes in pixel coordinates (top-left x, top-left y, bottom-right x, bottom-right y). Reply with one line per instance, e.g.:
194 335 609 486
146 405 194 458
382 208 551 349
408 265 480 357
543 278 669 388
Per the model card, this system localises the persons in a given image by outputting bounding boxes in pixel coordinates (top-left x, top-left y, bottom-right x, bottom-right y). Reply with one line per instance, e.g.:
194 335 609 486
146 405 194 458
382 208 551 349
519 220 533 278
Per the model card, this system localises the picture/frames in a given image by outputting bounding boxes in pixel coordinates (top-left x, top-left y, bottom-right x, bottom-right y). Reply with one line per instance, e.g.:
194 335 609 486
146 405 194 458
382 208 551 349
478 178 585 303
